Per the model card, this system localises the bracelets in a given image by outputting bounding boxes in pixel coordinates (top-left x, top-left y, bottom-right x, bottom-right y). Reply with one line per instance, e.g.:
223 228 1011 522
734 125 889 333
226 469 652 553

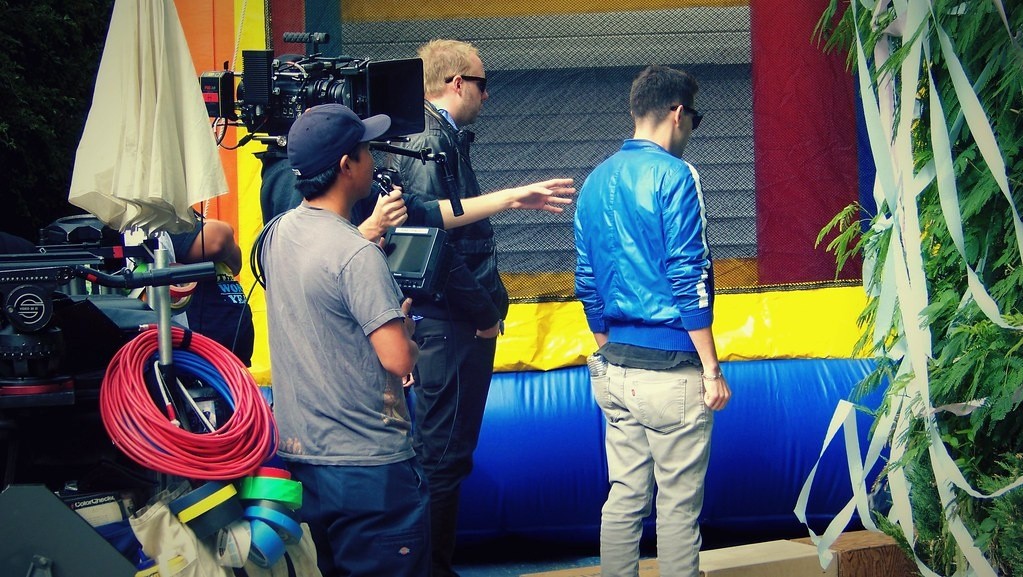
703 372 722 380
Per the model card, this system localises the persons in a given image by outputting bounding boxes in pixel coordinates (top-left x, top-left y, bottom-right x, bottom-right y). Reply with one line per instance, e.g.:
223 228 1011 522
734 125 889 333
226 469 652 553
382 40 509 577
100 216 257 371
261 104 433 577
573 63 732 577
260 55 576 464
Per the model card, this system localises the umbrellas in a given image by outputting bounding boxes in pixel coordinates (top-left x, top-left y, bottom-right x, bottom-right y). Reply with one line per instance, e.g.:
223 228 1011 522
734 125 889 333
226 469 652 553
68 0 230 364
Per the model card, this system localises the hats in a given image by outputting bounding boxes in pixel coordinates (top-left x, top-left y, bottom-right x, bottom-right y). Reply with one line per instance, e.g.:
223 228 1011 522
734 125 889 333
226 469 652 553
288 104 391 181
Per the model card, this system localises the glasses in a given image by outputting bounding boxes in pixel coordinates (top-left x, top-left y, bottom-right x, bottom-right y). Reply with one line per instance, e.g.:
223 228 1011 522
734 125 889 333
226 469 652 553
444 75 487 93
670 104 704 130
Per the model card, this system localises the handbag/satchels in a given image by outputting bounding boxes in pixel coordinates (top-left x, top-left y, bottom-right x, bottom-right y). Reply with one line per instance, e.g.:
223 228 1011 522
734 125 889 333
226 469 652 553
185 258 254 367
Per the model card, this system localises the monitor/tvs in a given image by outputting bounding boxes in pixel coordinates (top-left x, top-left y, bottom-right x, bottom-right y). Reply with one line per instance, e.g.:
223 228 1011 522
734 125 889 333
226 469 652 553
384 227 447 293
